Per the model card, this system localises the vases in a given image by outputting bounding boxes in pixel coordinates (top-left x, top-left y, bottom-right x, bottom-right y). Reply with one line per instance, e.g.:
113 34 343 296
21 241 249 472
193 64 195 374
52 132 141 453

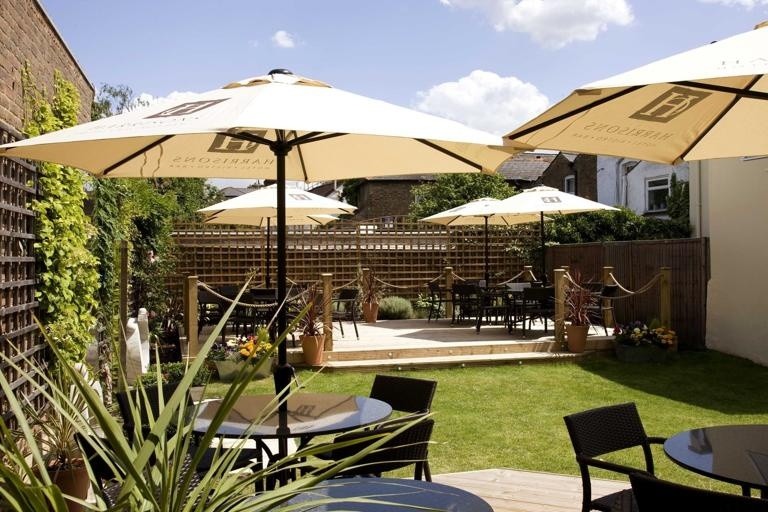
644 345 666 362
214 358 274 379
616 345 644 362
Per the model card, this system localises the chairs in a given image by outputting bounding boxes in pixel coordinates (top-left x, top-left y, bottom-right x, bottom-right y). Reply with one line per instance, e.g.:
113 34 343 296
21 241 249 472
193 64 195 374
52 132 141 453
518 287 550 335
198 290 226 341
428 282 452 321
333 288 359 337
233 293 265 337
505 282 543 325
332 419 435 480
627 471 765 512
117 383 257 475
73 433 124 492
562 402 667 512
249 288 276 311
306 374 438 482
451 284 477 324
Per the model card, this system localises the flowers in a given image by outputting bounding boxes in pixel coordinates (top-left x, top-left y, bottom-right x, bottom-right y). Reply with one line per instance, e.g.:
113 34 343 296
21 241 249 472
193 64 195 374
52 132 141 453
213 335 276 367
612 321 647 347
647 327 678 351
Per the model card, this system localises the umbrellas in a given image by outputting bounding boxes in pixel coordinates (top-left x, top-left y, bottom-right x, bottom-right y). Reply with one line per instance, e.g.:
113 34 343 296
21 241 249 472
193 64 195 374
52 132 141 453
203 215 340 288
506 22 768 167
484 184 622 286
417 195 554 291
0 69 535 487
196 182 358 218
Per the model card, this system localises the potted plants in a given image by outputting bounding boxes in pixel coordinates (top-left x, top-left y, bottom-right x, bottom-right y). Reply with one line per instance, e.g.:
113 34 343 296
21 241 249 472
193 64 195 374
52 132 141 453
553 283 598 354
17 356 96 512
287 282 333 364
358 270 383 323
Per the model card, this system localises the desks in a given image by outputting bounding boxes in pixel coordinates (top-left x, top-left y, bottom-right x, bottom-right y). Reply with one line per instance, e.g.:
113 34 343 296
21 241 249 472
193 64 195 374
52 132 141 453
169 393 392 488
662 424 768 499
248 477 494 512
